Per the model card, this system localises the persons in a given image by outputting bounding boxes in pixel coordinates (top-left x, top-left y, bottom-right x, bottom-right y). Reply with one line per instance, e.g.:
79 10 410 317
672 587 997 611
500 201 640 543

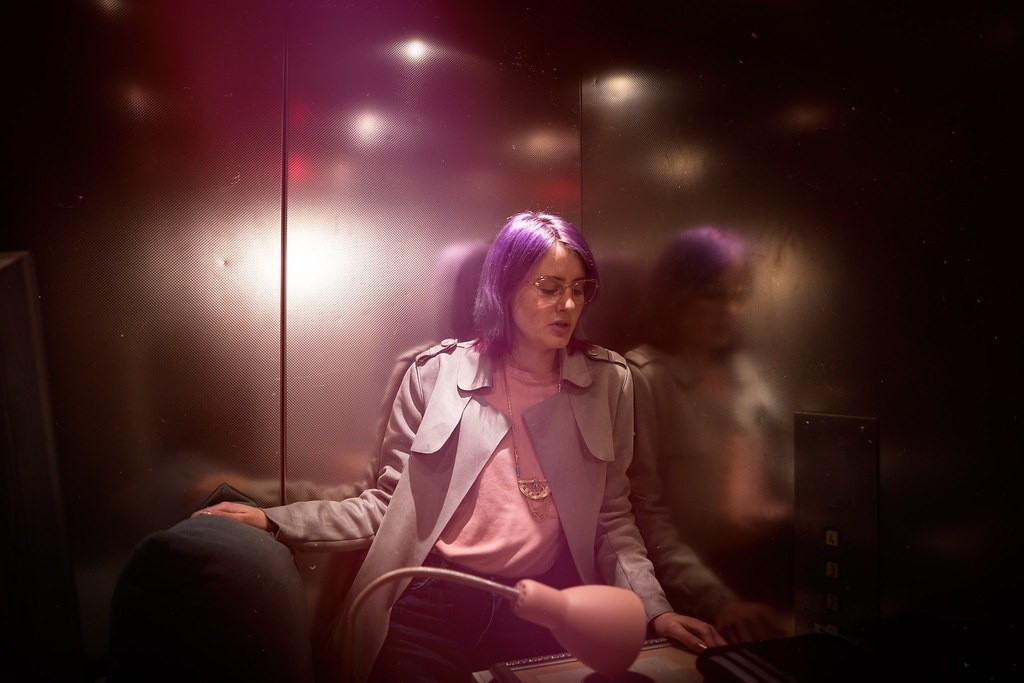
191 211 726 683
623 226 830 638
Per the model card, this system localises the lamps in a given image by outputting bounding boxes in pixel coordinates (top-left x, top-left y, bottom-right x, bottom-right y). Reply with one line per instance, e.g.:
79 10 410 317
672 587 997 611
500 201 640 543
342 566 646 683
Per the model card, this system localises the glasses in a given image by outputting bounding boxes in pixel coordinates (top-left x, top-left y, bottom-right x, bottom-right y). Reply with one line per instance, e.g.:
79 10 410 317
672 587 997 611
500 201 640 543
519 276 600 306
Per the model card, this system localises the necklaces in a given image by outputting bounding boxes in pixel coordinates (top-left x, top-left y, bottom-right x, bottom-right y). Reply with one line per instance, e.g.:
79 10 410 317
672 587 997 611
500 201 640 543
500 355 564 522
